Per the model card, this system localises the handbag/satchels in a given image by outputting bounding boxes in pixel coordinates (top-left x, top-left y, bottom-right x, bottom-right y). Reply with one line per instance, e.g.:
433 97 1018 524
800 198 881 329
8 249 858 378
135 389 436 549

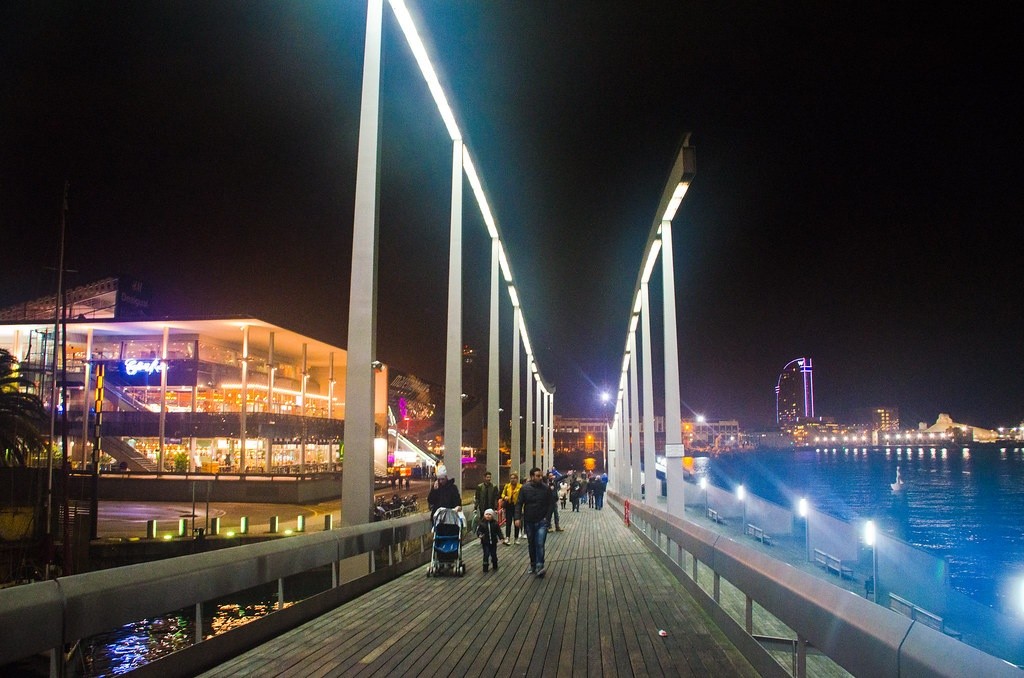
500 484 509 509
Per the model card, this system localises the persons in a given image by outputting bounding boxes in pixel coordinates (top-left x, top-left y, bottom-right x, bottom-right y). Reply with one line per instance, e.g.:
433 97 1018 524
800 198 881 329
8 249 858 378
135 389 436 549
473 471 500 545
476 509 504 571
515 467 553 575
428 465 462 568
391 474 409 490
501 471 529 545
542 466 608 533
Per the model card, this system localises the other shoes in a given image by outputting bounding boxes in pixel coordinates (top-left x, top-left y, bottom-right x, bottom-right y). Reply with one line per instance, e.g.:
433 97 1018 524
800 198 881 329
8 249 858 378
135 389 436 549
503 537 511 546
493 562 498 569
524 534 528 538
547 528 553 533
514 540 520 545
519 534 522 538
528 563 545 575
482 566 488 572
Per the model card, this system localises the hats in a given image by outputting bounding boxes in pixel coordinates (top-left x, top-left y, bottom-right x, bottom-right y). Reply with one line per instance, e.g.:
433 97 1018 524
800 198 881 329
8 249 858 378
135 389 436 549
484 509 495 518
437 465 447 477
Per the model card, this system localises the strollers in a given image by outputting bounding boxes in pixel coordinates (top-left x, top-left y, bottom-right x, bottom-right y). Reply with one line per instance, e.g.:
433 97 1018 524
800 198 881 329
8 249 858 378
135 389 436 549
426 508 466 577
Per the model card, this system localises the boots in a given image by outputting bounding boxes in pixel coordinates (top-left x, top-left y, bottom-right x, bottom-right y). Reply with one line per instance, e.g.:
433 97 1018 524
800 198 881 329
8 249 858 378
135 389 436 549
556 526 564 531
